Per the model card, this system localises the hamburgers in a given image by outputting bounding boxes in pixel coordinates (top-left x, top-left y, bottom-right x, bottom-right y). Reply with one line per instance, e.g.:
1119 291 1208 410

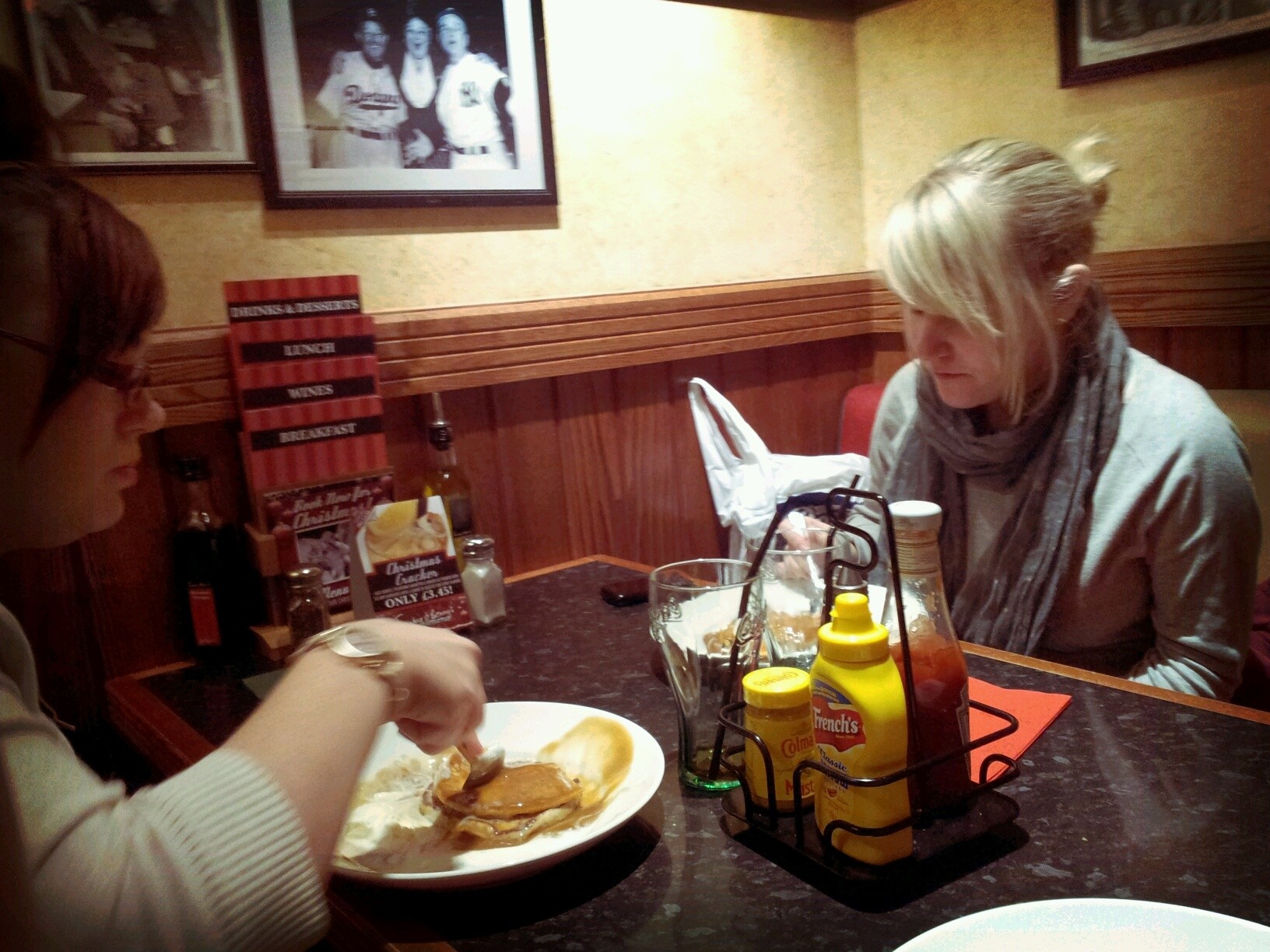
428 720 635 850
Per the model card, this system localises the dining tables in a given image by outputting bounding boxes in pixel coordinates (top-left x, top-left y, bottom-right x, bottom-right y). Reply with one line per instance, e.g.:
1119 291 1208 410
100 553 1270 951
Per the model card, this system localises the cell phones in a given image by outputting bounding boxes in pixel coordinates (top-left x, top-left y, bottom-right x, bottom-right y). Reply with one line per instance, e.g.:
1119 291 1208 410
600 570 696 608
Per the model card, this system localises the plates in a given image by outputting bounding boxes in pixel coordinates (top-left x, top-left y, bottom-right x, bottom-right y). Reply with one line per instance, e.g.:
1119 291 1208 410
330 699 668 889
870 898 1270 952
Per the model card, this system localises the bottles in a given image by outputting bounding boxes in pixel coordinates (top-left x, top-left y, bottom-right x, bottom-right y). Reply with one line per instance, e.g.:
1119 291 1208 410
282 567 330 646
424 420 475 573
168 458 239 669
462 538 509 626
878 501 973 814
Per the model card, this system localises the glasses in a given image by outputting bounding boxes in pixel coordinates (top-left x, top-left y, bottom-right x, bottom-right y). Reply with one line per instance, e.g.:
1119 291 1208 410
1 329 154 392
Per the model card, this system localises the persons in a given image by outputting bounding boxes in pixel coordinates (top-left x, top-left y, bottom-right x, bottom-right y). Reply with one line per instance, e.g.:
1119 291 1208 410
771 137 1264 704
0 158 490 952
329 9 500 169
31 0 188 152
132 0 222 152
306 7 414 170
402 6 518 170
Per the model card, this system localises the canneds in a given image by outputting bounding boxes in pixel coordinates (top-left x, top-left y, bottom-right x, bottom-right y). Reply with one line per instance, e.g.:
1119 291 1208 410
742 667 818 815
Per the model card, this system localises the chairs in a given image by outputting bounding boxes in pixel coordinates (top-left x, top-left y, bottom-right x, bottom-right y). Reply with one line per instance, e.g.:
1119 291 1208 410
1203 385 1270 715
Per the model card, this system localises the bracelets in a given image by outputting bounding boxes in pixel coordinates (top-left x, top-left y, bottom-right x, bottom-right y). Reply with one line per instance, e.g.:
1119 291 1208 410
288 623 410 723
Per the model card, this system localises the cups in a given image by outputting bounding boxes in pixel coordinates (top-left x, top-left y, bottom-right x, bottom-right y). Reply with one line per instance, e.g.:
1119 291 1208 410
648 559 766 790
747 530 857 671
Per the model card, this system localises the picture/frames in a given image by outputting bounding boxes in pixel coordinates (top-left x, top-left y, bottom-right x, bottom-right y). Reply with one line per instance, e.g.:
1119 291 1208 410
1054 0 1270 88
247 0 561 210
11 1 266 174
